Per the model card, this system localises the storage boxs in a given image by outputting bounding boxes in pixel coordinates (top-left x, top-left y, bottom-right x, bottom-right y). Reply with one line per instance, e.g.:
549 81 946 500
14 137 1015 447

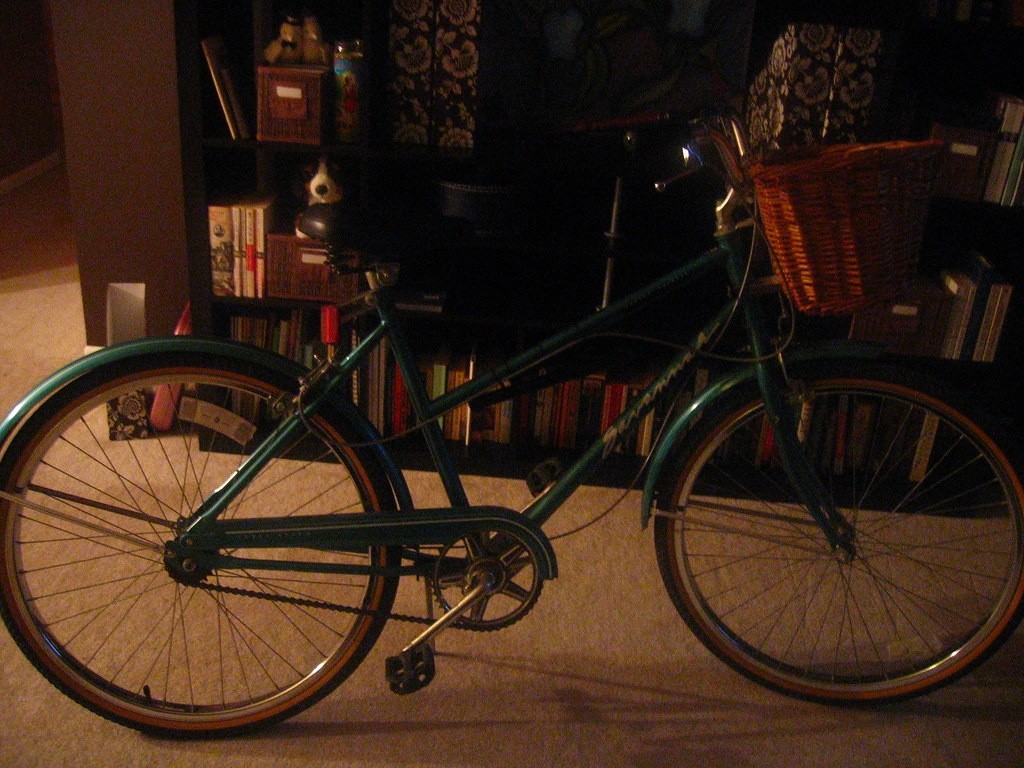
267 234 332 300
106 390 148 441
385 0 484 147
743 24 881 158
258 65 332 145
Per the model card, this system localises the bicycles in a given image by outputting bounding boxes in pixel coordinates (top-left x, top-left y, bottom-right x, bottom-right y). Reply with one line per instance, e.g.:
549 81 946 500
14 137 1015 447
0 91 1024 735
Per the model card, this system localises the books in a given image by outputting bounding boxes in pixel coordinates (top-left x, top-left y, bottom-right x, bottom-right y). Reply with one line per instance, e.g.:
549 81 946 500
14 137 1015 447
208 198 276 299
230 308 940 483
847 93 1024 363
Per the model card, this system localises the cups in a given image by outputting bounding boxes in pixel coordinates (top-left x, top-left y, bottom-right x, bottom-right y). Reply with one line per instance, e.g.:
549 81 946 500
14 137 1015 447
334 38 372 143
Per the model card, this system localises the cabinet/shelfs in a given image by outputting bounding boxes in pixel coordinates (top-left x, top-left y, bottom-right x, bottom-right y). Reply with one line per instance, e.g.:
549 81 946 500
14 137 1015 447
173 0 1024 518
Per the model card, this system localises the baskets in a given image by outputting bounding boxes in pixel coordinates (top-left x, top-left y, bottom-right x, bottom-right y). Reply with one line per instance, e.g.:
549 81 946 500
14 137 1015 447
749 138 946 317
